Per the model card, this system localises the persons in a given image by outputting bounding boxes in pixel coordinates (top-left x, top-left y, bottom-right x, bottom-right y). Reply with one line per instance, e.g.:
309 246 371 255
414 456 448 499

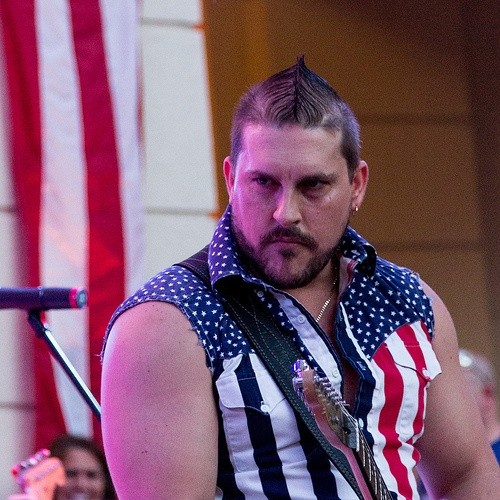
454 350 500 458
100 54 500 500
12 434 115 500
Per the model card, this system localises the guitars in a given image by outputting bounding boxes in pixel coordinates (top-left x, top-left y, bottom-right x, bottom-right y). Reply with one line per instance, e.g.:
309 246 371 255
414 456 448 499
292 358 394 500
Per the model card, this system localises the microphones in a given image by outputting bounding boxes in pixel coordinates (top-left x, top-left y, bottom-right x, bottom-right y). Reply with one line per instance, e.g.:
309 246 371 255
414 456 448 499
0 285 87 312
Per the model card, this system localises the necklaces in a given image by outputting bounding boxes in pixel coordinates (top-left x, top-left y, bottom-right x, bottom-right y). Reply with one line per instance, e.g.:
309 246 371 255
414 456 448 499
310 253 338 324
